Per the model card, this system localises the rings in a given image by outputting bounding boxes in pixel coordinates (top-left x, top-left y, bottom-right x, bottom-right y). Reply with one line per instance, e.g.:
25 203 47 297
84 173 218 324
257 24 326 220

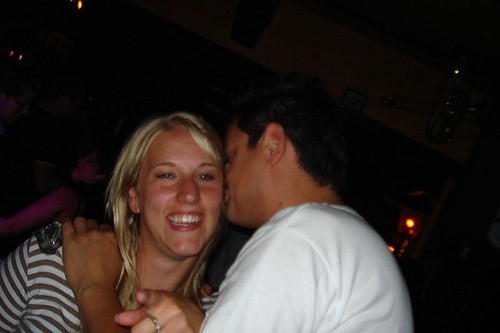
147 315 161 333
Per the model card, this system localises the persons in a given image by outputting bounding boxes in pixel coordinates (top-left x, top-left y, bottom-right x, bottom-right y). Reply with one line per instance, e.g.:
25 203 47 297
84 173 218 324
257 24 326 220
60 83 415 333
1 114 225 333
